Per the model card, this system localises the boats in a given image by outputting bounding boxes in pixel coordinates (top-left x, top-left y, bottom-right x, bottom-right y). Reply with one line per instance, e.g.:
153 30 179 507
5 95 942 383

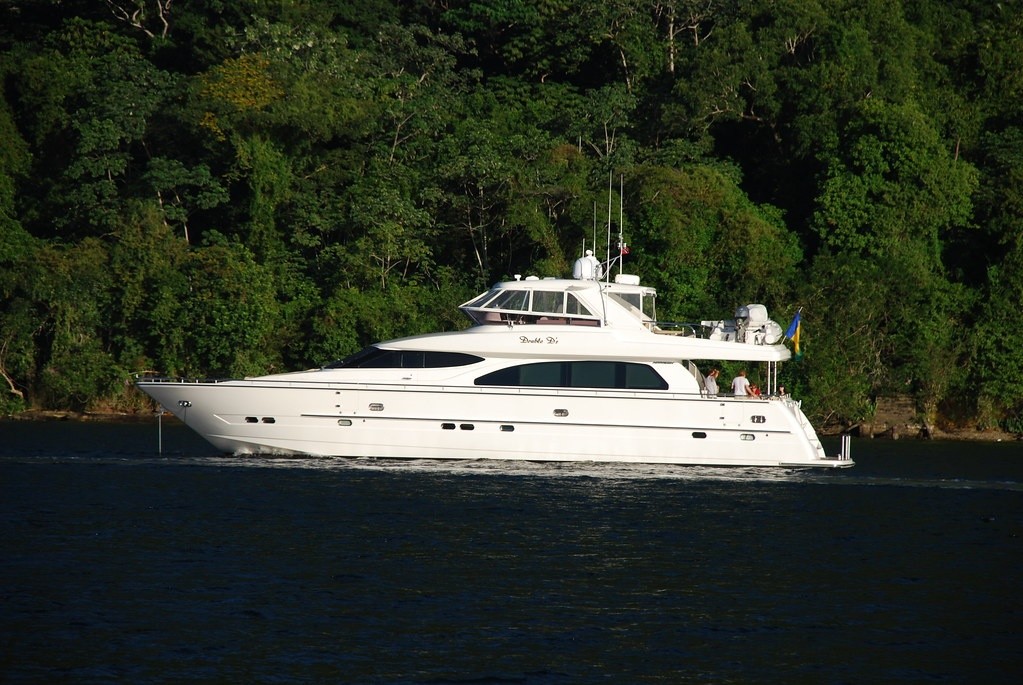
135 169 856 469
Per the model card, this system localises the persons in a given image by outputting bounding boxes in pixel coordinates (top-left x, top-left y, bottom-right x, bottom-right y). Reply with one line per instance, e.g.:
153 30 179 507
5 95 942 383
705 368 719 397
731 369 762 397
778 385 787 395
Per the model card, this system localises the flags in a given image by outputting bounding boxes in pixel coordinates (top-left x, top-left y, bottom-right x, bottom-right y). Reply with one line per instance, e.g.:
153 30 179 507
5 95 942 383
786 310 802 361
